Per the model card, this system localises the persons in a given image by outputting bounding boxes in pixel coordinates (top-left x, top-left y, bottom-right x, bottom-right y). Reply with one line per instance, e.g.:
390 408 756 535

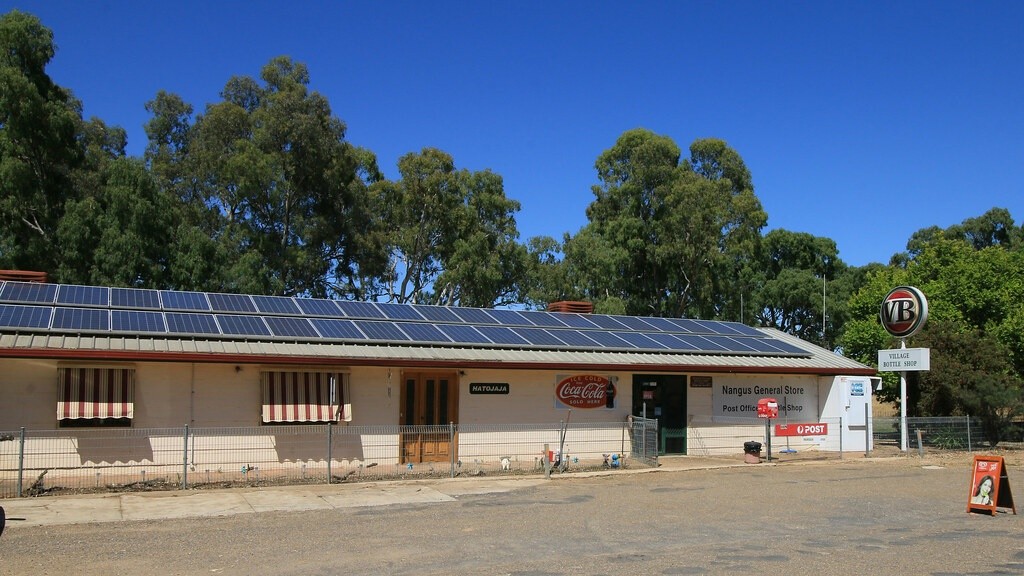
971 476 994 507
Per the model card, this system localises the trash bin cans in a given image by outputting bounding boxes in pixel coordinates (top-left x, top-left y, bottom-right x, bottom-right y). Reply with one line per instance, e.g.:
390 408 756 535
744 441 763 464
